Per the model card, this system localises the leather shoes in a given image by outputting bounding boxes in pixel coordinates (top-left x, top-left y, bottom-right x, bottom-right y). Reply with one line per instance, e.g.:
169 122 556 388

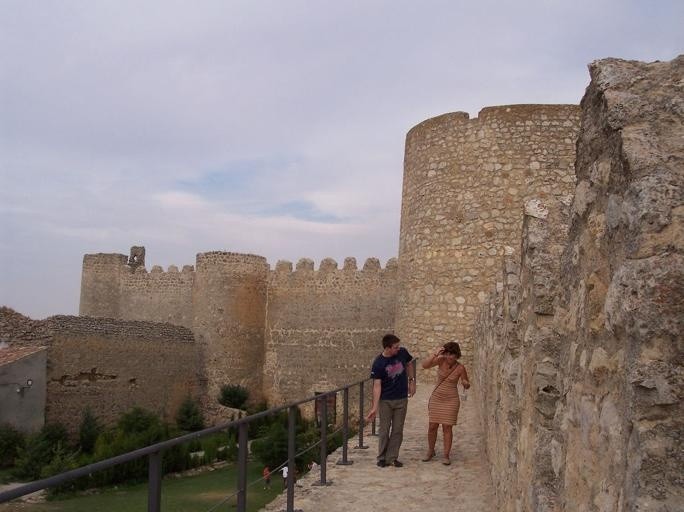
377 459 403 467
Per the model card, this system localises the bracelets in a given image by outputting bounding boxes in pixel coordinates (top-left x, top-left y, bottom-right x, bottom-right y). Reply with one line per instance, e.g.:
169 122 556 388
433 352 438 357
409 378 415 381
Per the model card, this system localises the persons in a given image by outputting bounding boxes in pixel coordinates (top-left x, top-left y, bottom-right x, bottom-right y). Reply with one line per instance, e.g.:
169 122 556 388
420 342 471 465
367 334 417 467
279 465 288 489
308 460 317 469
262 464 272 491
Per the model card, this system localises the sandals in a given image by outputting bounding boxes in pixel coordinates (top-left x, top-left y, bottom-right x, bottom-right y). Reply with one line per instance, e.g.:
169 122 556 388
442 457 451 465
423 451 436 462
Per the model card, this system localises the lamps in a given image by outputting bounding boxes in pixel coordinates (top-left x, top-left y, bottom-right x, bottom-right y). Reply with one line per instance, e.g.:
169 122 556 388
21 379 33 392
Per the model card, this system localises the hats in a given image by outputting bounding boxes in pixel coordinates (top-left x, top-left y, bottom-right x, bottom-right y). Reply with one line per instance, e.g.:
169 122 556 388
441 342 461 359
